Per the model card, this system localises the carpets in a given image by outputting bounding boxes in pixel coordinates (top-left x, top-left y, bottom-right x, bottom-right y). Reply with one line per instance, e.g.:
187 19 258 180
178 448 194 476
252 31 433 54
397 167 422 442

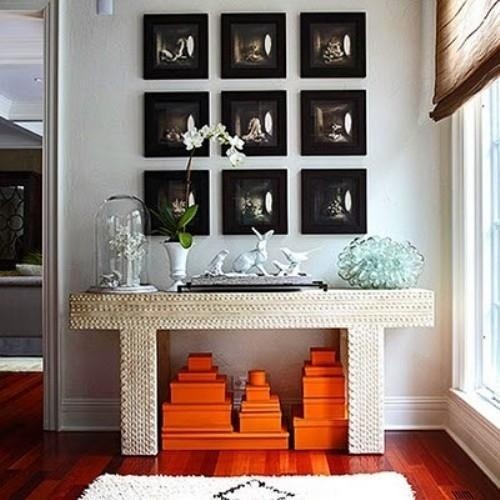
78 471 417 500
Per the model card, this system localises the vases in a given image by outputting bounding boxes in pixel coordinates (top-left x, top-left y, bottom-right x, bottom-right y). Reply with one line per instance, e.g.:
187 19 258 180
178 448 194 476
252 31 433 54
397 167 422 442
163 239 195 292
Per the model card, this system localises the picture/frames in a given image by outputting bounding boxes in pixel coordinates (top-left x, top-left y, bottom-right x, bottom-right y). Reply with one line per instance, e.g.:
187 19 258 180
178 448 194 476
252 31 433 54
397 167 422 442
222 167 288 236
141 169 210 238
300 167 367 236
143 11 210 81
220 90 288 157
300 89 367 157
219 11 287 81
143 90 210 158
299 11 367 81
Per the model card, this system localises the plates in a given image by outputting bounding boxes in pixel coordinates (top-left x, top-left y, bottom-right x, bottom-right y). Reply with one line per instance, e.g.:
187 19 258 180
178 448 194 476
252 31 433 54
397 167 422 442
15 264 42 276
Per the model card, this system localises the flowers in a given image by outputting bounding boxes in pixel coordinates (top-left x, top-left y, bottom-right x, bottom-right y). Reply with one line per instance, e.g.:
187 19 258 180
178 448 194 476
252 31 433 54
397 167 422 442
145 115 245 248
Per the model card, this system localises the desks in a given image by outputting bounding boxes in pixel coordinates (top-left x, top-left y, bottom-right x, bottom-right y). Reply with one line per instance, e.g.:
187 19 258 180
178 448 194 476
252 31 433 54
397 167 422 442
68 285 435 457
